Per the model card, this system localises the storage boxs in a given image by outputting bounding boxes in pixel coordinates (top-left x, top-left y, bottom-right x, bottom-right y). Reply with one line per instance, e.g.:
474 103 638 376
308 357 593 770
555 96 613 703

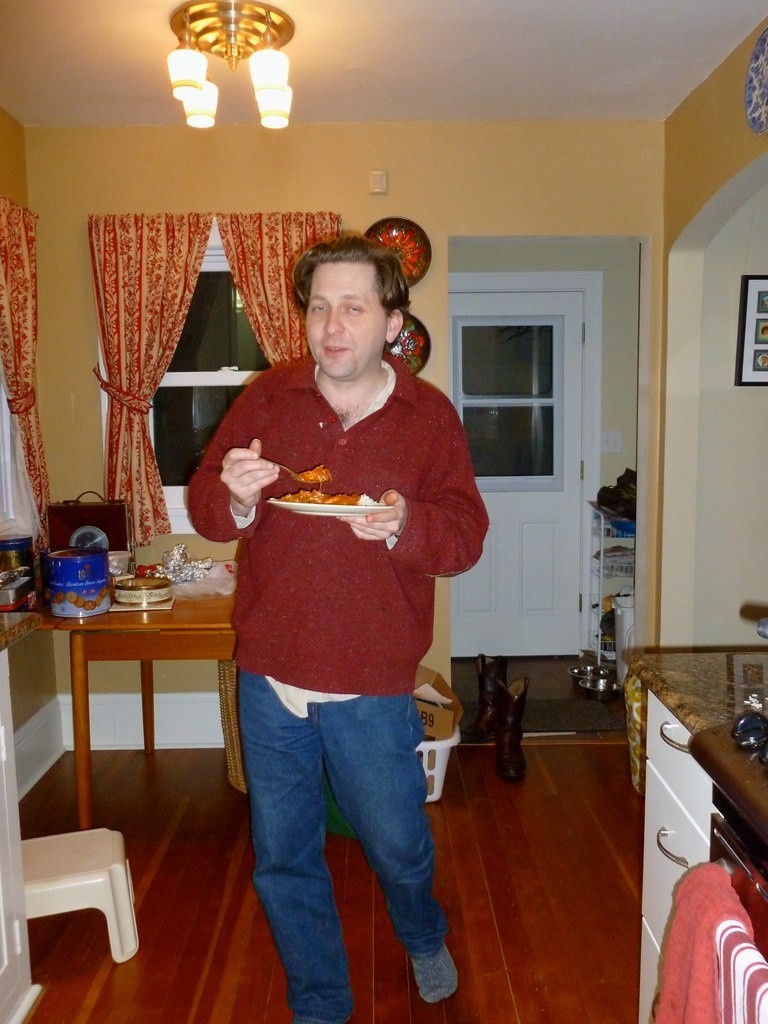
411 662 467 805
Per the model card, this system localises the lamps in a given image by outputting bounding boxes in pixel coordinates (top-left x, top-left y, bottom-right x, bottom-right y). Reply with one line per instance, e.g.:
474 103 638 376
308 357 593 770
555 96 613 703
164 0 293 131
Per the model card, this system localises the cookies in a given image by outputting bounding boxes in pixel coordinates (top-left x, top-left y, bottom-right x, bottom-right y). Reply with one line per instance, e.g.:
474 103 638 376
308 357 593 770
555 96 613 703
53 588 107 609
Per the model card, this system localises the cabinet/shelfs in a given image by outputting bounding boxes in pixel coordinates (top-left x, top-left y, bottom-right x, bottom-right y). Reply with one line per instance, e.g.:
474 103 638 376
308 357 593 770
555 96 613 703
626 692 723 1024
587 506 635 667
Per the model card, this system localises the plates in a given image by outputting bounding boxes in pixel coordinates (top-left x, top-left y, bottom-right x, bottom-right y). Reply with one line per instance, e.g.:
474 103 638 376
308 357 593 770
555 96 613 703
384 312 432 376
267 499 394 516
364 216 431 288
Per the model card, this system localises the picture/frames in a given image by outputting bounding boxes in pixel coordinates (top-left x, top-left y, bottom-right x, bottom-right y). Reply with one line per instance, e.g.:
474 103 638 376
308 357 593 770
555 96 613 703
733 273 768 387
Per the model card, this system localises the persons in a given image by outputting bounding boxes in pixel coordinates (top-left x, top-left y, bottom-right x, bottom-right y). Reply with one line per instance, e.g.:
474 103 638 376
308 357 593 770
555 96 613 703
190 236 490 1024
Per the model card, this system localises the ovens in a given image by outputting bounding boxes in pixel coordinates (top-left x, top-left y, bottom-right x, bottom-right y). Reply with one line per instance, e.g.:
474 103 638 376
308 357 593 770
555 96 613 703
709 815 768 964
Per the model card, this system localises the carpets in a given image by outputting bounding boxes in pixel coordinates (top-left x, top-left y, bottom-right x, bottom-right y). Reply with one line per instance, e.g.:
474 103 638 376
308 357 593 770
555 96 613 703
459 694 626 735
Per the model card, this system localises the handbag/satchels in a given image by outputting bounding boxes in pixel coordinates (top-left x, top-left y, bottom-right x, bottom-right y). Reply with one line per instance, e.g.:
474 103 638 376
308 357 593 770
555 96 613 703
598 467 637 521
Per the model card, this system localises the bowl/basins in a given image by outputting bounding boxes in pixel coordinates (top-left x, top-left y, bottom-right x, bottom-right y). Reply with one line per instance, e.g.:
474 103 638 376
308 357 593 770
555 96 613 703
578 678 622 699
567 665 610 680
108 551 131 576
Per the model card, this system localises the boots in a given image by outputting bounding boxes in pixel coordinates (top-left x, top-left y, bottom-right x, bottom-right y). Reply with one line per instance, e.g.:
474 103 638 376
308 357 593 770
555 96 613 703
458 654 508 743
494 675 525 776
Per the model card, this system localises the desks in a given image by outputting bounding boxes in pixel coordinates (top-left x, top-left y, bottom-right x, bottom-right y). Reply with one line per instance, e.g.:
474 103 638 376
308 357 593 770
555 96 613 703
58 592 236 832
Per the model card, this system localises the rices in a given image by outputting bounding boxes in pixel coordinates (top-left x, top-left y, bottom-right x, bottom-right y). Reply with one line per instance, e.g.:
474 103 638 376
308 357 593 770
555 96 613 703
356 493 378 505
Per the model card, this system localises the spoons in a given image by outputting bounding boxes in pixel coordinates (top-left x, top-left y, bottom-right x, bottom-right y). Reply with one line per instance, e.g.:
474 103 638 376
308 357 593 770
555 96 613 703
258 457 324 482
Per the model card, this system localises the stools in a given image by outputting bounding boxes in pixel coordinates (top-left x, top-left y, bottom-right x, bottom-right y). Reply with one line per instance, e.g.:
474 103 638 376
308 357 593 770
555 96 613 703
21 825 144 965
